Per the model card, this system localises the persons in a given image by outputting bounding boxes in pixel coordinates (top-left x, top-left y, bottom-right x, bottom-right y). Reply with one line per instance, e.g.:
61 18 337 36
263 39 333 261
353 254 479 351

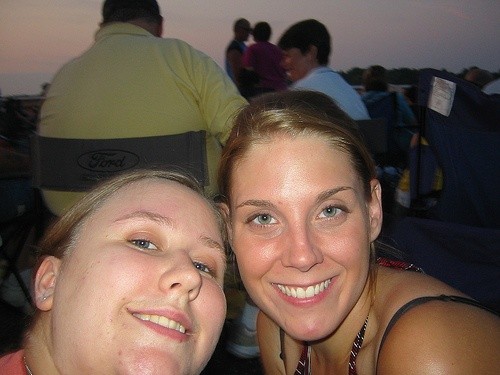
35 0 260 358
0 160 228 375
225 18 288 101
217 90 500 375
403 87 426 122
277 19 371 120
360 66 419 149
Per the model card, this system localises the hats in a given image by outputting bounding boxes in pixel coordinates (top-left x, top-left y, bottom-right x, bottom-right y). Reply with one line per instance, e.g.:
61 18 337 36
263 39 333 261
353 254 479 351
235 18 253 33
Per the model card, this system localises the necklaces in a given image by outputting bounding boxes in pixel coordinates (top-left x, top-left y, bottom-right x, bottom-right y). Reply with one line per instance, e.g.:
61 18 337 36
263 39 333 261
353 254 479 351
23 354 32 375
293 305 372 375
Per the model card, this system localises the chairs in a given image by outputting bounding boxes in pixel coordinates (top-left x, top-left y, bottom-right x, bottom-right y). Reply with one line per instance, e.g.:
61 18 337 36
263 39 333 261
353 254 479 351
14 130 210 225
